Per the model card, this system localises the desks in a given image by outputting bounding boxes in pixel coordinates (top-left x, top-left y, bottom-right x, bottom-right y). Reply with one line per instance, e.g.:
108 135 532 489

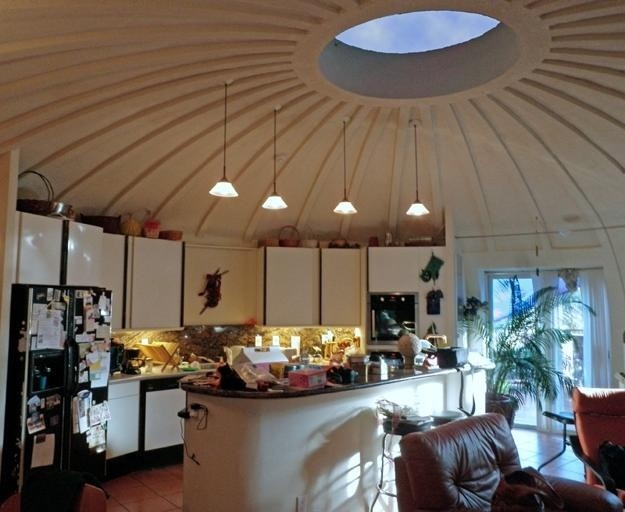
535 408 575 476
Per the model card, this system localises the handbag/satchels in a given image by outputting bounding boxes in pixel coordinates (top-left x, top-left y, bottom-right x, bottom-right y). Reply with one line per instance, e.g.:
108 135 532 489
491 467 564 512
599 439 624 490
437 348 469 368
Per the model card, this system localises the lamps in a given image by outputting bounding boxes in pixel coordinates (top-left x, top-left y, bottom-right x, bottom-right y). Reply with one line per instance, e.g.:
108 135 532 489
404 117 431 219
333 120 360 216
259 108 289 211
210 78 241 197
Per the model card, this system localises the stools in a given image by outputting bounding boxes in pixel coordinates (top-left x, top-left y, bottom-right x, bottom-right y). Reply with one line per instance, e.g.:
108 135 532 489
419 409 463 424
370 413 434 512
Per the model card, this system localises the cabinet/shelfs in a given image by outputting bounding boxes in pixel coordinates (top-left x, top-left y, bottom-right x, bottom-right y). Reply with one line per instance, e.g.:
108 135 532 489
321 249 360 326
130 237 182 329
266 247 320 328
368 245 419 295
105 382 138 460
12 211 103 288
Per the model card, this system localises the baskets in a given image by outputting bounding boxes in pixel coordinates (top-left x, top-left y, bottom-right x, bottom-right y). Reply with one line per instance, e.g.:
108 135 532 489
404 240 434 246
16 166 54 216
258 226 318 247
78 209 183 240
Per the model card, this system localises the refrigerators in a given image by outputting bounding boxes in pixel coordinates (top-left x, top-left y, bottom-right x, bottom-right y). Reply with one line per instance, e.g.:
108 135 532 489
0 283 113 505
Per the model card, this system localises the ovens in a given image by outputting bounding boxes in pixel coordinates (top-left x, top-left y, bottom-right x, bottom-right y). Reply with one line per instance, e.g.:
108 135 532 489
365 290 420 345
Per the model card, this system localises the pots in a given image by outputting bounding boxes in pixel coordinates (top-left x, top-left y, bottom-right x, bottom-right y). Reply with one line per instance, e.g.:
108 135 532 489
77 212 122 233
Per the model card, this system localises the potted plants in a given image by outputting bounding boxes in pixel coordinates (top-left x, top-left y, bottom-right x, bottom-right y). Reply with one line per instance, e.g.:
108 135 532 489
458 272 599 427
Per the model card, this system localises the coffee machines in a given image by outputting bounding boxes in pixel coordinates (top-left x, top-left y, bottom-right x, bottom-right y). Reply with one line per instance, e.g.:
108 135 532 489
121 349 141 375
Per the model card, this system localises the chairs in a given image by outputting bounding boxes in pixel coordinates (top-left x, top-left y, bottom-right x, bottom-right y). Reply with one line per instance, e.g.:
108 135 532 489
1 480 108 512
569 383 625 502
396 411 622 510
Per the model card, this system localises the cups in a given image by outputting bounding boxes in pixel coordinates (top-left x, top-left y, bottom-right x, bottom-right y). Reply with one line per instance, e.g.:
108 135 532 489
144 358 153 373
284 365 296 378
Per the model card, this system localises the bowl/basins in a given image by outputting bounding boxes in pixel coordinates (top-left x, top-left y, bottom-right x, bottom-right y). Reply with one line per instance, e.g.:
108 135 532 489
160 231 183 240
51 201 73 214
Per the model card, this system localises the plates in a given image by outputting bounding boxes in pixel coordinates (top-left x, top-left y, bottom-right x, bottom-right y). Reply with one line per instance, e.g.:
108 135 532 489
182 367 198 371
